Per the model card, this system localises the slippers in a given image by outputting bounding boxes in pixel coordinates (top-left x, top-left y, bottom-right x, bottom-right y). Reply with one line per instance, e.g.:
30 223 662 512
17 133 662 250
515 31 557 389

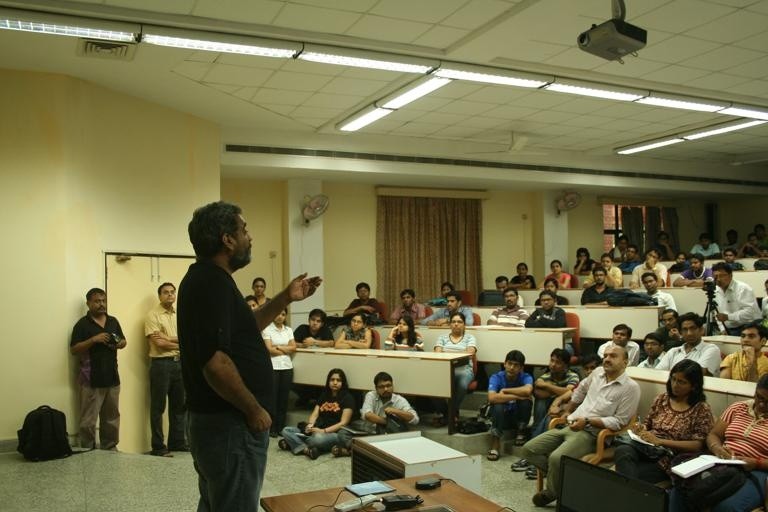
485 446 500 463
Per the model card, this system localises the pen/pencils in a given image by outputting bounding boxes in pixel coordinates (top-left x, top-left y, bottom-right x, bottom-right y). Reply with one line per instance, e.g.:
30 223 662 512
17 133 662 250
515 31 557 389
638 416 641 432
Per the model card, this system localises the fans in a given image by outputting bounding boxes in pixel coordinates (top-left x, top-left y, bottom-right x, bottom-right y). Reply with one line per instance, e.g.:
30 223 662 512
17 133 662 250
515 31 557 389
302 194 329 224
557 192 582 215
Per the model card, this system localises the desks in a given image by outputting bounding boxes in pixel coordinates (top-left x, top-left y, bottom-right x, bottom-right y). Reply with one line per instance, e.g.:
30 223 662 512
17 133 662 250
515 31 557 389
261 473 513 512
358 302 664 368
701 334 768 361
293 347 479 435
455 260 768 307
624 366 758 423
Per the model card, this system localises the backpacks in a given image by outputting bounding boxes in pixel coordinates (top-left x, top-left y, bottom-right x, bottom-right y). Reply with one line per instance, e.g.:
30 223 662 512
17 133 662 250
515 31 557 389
14 403 77 463
604 288 659 307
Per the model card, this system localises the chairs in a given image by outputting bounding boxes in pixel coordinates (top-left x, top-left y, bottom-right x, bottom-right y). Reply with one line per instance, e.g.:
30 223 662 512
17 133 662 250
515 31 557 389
536 412 638 493
555 455 669 512
609 464 671 489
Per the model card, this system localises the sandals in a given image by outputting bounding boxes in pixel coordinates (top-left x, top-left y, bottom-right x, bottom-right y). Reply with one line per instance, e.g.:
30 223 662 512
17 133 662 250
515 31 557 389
304 446 320 461
275 436 290 451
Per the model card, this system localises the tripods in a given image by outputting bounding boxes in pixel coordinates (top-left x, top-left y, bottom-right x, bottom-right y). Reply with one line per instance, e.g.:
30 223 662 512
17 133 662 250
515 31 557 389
696 300 734 335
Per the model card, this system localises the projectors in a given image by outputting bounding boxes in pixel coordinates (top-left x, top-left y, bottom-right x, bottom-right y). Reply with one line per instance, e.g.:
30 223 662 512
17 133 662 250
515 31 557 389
577 19 646 63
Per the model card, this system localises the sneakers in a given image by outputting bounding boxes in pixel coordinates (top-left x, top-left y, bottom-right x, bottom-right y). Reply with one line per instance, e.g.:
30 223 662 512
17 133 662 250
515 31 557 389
173 443 193 453
151 446 175 460
510 455 547 482
530 487 558 509
330 444 352 460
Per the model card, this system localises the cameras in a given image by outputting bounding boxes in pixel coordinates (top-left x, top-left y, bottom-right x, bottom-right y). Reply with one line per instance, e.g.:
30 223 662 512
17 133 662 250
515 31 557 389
703 278 720 294
104 329 123 346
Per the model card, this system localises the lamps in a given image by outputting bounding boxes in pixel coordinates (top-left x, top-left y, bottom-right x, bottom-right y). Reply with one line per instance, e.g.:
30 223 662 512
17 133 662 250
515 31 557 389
295 45 442 76
141 34 296 59
334 103 395 132
716 103 768 120
544 78 650 102
683 120 768 141
0 19 137 44
375 74 452 110
614 135 686 155
431 61 555 89
633 90 732 113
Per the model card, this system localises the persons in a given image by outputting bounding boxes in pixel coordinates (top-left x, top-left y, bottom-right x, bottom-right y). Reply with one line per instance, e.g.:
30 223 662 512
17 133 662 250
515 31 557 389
178 202 323 510
70 289 126 450
145 284 190 455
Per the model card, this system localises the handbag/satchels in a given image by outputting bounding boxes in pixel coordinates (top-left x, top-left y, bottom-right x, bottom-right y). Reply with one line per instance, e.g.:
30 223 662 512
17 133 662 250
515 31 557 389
374 414 408 435
673 453 749 512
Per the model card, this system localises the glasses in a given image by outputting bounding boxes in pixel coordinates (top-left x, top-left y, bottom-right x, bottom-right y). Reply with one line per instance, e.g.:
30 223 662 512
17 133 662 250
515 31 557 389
679 326 699 332
641 342 662 348
754 390 767 404
375 384 392 390
668 374 690 386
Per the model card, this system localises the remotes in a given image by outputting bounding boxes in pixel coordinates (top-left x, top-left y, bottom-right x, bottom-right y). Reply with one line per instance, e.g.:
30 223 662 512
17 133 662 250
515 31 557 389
334 494 378 511
